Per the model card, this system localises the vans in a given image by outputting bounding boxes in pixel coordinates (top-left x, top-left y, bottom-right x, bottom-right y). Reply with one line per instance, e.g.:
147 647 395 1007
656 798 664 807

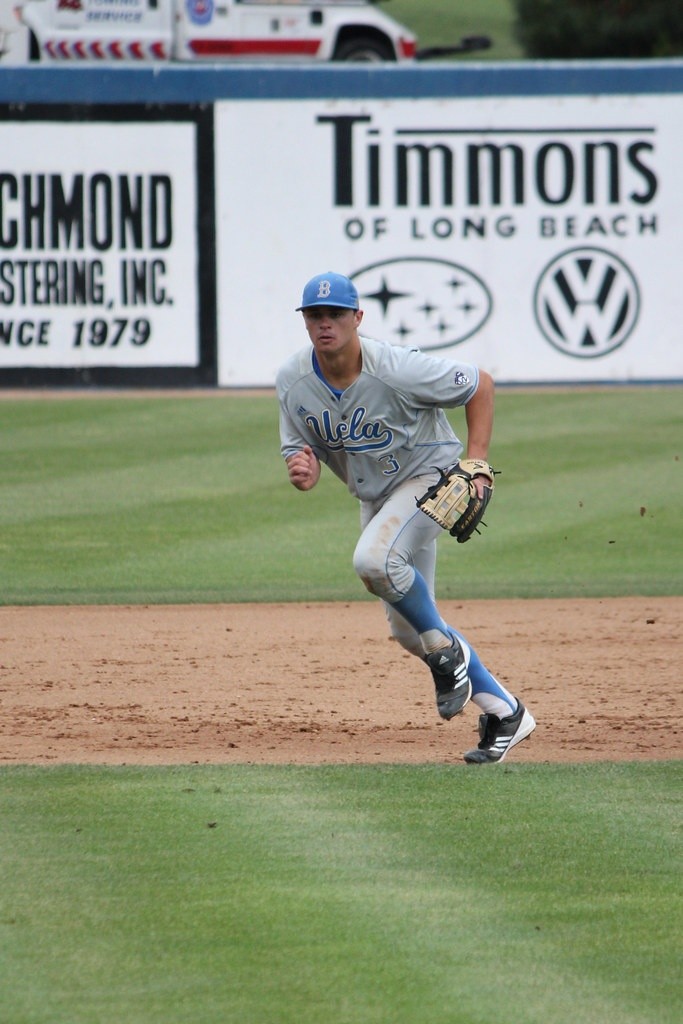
0 0 418 70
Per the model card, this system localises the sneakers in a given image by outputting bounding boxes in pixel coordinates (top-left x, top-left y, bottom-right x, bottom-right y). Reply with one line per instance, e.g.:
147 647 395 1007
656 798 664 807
463 697 536 764
424 633 472 719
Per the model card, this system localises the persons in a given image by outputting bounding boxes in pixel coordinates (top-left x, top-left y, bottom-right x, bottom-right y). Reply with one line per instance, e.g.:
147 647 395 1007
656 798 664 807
276 272 536 767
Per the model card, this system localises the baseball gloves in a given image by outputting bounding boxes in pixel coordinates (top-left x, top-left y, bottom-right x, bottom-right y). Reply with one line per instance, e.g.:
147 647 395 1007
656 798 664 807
414 459 502 543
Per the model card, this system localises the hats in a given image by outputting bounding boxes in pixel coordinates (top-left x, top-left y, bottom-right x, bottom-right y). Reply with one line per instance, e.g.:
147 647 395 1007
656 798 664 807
296 272 359 312
